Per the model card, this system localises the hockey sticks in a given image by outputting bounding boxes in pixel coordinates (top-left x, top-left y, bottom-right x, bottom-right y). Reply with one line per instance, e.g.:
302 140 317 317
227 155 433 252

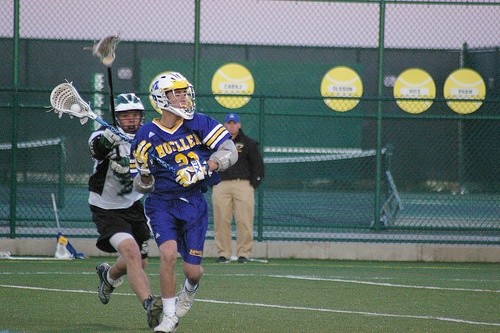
50 193 70 259
50 82 178 174
0 253 72 260
92 33 121 160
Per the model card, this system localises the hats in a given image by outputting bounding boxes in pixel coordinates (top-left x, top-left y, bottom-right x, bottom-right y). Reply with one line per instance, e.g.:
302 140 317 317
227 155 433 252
225 112 240 124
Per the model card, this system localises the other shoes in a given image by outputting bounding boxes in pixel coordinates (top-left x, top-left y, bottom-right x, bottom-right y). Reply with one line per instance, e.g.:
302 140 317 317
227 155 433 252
215 257 230 263
238 256 252 264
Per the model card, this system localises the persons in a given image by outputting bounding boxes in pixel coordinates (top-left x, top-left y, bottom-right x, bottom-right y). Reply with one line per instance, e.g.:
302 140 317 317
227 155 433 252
89 92 162 328
210 112 264 264
130 71 239 333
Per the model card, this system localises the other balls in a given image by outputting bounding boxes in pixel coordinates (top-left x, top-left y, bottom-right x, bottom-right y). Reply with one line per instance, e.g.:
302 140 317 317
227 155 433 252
70 103 82 113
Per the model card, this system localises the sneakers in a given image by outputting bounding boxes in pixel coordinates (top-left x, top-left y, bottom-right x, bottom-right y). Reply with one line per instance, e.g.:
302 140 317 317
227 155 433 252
154 312 179 333
146 295 163 329
96 263 115 304
176 279 201 317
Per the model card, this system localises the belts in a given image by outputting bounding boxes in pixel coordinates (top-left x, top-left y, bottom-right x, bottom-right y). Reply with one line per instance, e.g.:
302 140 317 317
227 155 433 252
232 177 249 180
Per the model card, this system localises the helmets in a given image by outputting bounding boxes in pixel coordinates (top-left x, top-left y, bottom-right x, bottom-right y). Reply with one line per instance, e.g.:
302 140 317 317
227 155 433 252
148 71 195 120
111 92 145 140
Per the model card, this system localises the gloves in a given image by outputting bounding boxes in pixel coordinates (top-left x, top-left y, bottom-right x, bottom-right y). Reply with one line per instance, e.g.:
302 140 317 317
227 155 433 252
250 176 262 187
175 159 212 192
99 125 126 149
110 153 133 182
132 140 158 176
